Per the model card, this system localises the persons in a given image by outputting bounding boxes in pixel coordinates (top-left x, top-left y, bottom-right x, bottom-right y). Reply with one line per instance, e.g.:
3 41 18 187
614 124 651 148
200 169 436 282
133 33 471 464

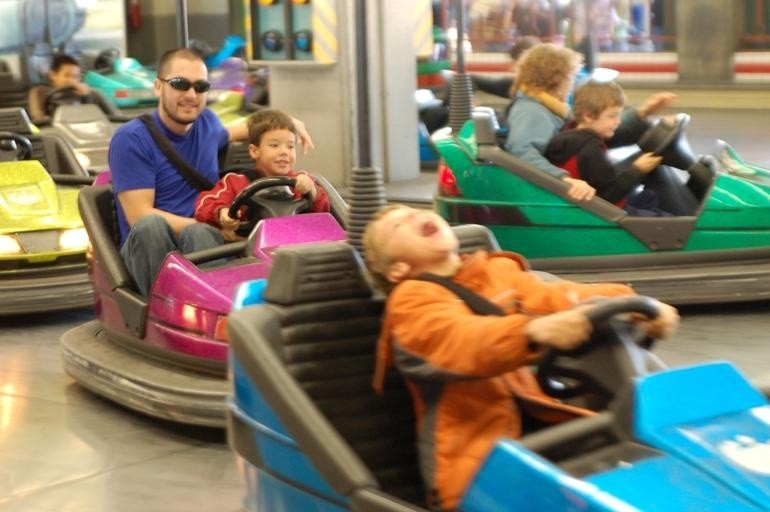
542 77 674 216
503 43 680 204
28 55 95 126
107 50 316 300
363 202 680 509
194 109 331 232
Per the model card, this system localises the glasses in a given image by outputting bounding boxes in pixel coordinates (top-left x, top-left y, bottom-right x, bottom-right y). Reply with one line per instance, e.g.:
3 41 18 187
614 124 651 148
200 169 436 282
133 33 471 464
159 77 211 93
